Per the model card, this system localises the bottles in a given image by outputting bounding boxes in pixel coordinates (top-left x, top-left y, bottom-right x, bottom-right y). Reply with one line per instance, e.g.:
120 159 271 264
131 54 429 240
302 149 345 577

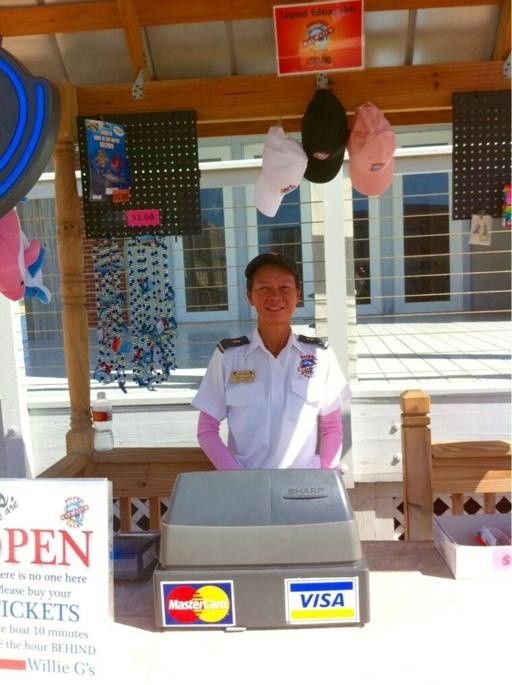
90 390 115 452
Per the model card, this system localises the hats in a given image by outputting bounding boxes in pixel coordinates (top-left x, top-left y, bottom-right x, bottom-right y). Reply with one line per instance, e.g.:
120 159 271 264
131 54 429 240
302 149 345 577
348 102 396 195
301 90 348 182
254 126 308 217
246 252 298 276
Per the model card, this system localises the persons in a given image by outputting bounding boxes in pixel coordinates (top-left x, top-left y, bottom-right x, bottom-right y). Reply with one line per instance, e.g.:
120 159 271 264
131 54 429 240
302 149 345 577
194 253 350 476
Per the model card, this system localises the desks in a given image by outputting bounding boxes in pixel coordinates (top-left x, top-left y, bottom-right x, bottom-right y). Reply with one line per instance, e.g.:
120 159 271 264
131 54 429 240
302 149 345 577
109 540 454 685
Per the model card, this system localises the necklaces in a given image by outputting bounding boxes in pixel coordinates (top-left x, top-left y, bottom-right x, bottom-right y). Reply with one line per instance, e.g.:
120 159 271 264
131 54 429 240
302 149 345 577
89 230 184 394
